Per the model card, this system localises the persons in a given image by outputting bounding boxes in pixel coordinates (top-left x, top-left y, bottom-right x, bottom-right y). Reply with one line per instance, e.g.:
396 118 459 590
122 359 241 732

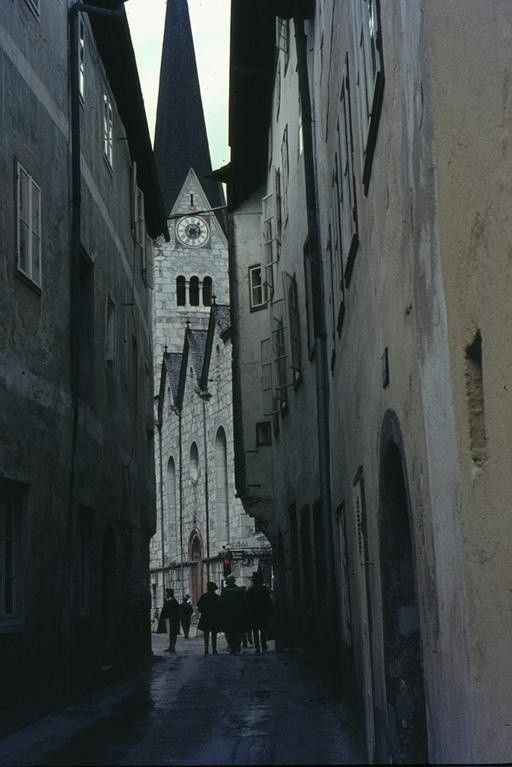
162 570 272 655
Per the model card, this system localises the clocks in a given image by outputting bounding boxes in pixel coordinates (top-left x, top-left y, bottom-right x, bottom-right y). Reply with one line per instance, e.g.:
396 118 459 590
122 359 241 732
174 214 212 249
